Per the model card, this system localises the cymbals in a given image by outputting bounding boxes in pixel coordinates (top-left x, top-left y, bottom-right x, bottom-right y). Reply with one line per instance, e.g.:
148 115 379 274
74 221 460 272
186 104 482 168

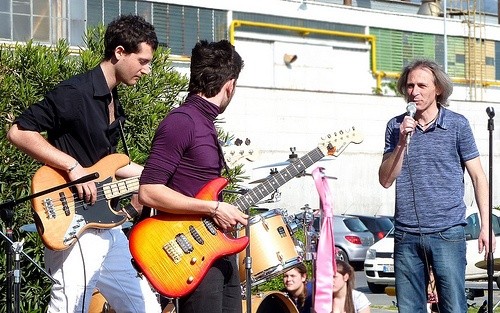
20 219 134 233
253 155 335 170
250 168 282 184
475 257 500 272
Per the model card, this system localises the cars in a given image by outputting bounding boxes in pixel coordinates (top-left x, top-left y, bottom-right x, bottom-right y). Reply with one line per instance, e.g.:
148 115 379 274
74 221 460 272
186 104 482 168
364 208 500 294
346 213 395 242
290 213 376 265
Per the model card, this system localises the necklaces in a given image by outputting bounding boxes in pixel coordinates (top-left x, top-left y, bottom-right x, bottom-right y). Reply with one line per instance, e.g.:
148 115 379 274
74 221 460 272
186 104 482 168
413 108 439 127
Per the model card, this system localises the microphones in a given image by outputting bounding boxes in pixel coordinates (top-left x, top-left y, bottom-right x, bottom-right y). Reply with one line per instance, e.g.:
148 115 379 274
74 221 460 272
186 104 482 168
406 102 417 144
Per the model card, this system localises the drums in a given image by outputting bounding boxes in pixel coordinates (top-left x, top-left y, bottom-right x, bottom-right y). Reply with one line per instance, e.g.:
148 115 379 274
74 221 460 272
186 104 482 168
237 208 303 291
88 288 115 313
160 291 299 313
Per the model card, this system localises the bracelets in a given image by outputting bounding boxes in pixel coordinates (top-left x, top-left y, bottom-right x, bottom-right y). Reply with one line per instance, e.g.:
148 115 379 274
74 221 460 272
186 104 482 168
67 161 79 173
210 200 219 217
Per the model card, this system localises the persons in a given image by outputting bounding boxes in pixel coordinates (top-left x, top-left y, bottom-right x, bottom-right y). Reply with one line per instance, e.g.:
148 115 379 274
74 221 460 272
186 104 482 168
280 262 310 313
5 13 163 313
139 40 251 313
378 58 496 313
331 259 371 313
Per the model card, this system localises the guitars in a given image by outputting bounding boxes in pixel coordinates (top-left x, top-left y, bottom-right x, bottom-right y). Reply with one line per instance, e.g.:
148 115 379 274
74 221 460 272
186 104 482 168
127 123 363 297
30 131 255 252
